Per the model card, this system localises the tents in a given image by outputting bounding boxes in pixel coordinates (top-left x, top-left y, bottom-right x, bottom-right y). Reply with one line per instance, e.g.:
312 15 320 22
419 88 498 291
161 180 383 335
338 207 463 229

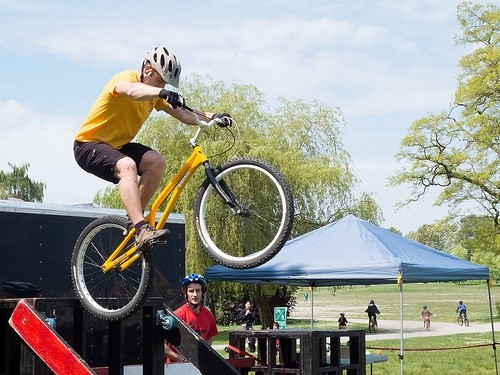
203 213 499 375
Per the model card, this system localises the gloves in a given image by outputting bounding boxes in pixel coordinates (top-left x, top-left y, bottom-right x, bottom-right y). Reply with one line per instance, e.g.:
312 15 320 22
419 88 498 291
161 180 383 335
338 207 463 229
159 89 179 109
212 113 233 127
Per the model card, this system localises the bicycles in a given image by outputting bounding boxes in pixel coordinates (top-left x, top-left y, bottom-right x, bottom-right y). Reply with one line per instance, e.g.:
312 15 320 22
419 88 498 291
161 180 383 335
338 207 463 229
69 94 295 321
421 313 434 331
367 311 380 333
456 312 469 327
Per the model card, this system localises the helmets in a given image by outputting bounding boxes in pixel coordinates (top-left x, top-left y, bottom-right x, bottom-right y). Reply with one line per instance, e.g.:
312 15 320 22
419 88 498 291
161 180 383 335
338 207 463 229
181 273 207 292
144 45 182 88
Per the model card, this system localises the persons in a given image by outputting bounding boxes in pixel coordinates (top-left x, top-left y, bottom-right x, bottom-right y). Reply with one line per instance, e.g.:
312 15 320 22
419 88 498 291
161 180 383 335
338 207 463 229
420 306 433 328
456 301 467 322
241 301 256 352
304 291 308 304
273 322 280 356
161 274 218 363
337 313 348 326
72 45 231 250
366 300 380 326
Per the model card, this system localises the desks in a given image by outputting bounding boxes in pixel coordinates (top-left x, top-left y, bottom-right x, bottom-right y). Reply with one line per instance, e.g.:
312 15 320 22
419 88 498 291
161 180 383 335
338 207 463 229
326 355 387 375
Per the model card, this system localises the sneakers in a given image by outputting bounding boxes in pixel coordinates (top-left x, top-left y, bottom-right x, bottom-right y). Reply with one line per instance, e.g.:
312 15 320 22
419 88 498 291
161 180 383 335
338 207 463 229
134 224 170 255
